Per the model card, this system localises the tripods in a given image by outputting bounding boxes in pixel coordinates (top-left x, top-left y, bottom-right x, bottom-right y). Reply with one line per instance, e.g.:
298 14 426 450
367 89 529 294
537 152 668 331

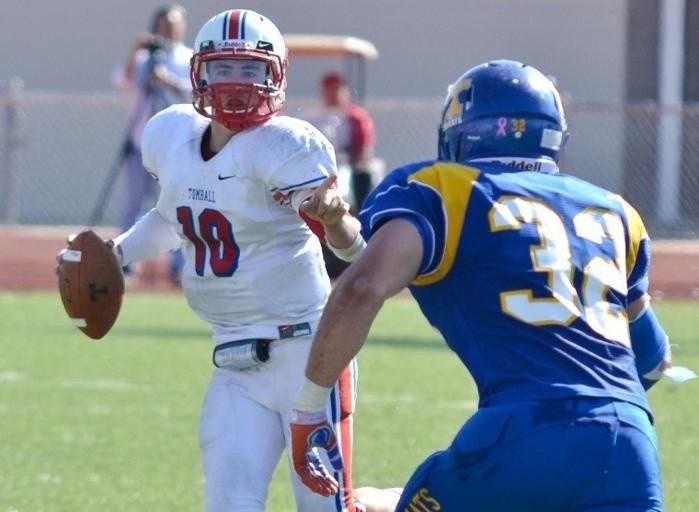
83 81 171 294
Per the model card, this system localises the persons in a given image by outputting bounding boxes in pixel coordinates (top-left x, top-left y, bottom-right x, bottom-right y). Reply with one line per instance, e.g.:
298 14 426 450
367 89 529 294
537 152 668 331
55 6 371 511
285 51 675 511
106 3 202 292
318 71 371 280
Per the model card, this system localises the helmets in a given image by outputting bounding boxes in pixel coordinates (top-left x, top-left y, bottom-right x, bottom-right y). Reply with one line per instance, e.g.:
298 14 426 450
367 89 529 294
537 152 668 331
190 8 289 132
437 59 570 176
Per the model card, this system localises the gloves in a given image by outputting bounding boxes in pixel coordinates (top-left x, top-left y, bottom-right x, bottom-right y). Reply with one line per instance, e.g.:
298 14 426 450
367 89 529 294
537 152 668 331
288 409 346 497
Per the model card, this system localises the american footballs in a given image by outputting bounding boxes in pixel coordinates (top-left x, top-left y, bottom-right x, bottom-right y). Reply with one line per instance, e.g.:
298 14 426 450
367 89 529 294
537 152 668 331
56 231 124 340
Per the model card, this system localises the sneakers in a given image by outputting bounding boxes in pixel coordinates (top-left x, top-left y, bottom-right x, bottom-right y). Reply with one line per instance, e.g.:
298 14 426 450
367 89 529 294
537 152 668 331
353 486 406 512
